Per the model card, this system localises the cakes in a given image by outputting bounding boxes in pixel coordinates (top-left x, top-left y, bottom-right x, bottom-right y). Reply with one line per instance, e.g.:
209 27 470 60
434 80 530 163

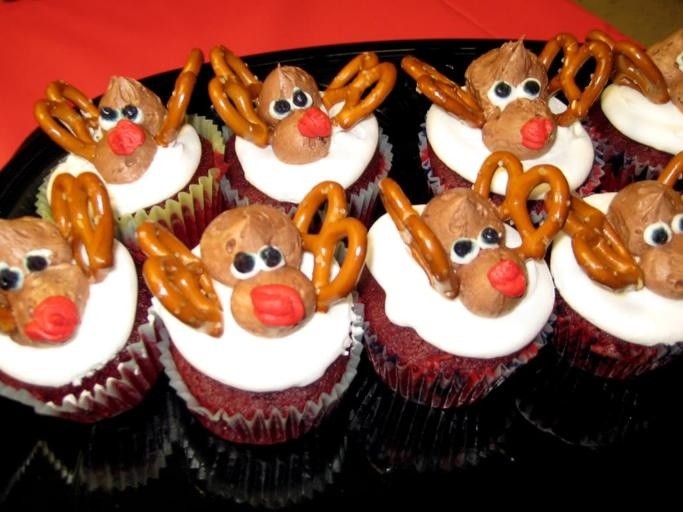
544 152 683 376
0 173 161 421
585 25 683 183
35 48 227 259
138 182 367 432
208 44 396 237
359 152 570 407
402 33 613 222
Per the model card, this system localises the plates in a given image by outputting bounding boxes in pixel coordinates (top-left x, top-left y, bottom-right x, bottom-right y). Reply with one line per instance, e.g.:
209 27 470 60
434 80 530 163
0 38 683 508
